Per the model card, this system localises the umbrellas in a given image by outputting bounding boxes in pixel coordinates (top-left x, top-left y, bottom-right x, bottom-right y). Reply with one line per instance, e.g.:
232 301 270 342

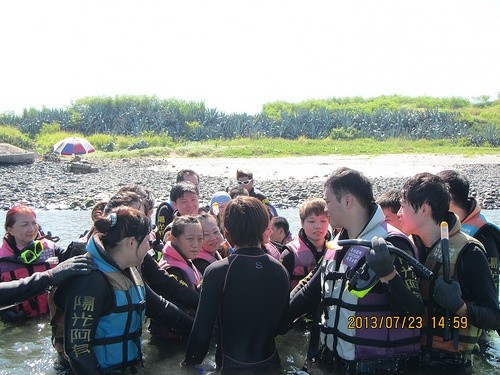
52 135 94 159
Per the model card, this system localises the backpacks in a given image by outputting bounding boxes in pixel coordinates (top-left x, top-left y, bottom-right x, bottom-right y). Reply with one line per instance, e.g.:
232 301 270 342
46 265 80 360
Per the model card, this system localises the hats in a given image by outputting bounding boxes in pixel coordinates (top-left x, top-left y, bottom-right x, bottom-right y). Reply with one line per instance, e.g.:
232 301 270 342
209 191 230 206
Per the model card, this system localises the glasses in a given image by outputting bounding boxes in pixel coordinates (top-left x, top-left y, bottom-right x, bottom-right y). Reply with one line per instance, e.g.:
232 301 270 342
237 179 253 185
18 241 45 262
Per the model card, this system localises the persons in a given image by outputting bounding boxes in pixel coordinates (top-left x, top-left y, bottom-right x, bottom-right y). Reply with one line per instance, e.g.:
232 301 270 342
395 172 500 375
0 255 98 307
380 199 406 237
49 170 296 375
434 168 500 371
2 205 66 323
284 167 426 375
289 200 335 287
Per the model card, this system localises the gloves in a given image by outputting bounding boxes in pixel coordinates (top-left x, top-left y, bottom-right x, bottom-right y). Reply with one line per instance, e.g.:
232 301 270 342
433 274 466 313
366 236 398 277
49 254 98 282
